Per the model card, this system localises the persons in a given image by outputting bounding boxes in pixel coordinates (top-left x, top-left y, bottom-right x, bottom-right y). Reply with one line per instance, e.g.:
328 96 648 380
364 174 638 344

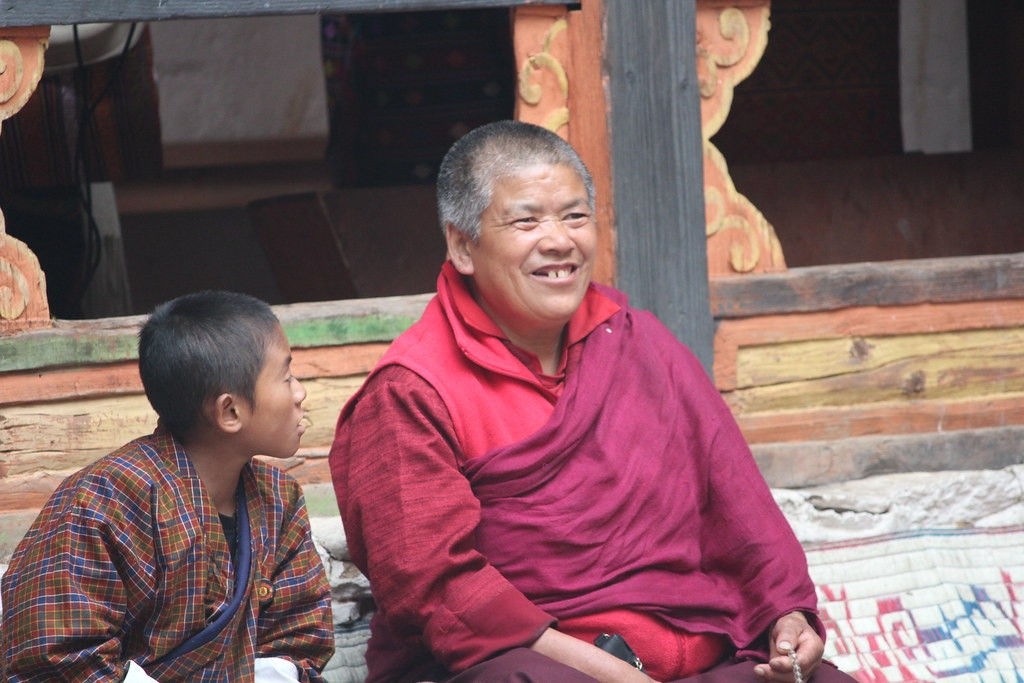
0 290 335 683
326 118 860 683
